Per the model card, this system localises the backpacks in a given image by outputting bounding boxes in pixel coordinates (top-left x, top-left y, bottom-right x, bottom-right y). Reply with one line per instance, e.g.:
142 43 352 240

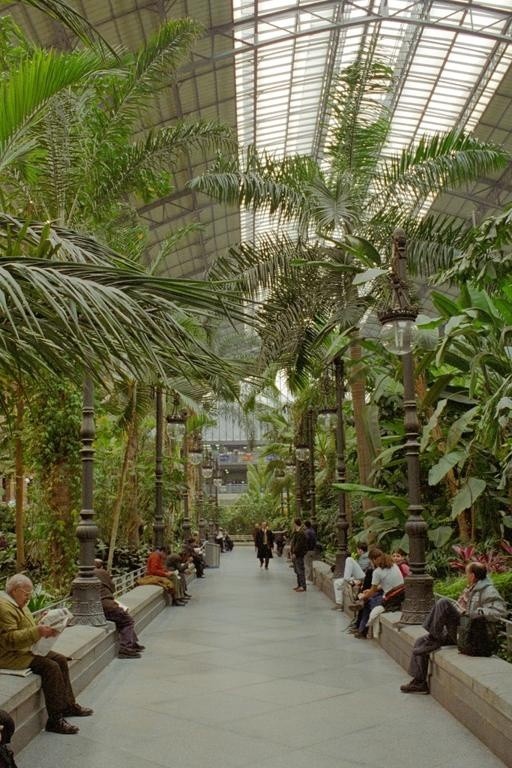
455 608 499 658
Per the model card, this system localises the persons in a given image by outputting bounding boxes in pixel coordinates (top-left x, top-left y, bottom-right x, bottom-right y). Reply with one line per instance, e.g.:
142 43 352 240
399 563 508 695
93 557 146 660
254 516 316 594
142 529 207 606
337 539 413 640
215 523 233 551
1 710 18 767
1 572 93 735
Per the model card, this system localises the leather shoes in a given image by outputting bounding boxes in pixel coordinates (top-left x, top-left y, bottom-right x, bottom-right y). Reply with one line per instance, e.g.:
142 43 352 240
45 717 80 734
63 702 94 717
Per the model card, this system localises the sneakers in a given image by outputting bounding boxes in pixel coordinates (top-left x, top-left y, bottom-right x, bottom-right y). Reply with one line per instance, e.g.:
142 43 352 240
134 642 146 651
348 600 366 612
400 677 430 693
292 585 306 593
118 648 141 659
411 639 441 656
172 591 192 607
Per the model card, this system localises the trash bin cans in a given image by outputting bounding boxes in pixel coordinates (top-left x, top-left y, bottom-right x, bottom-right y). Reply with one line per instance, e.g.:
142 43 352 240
205 543 221 568
